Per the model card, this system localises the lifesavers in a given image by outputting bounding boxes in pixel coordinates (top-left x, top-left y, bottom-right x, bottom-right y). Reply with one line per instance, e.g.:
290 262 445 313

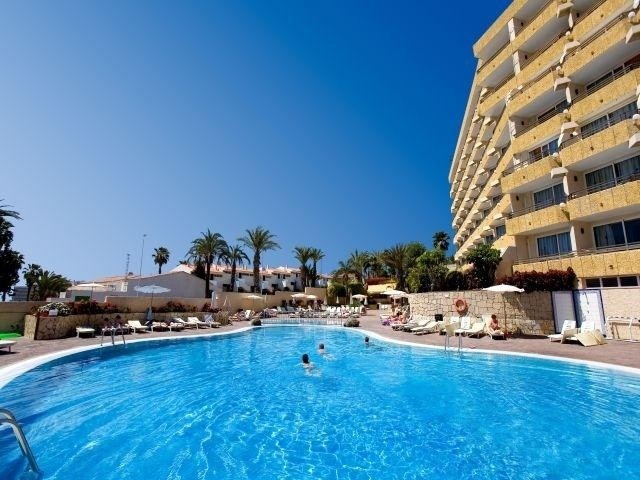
454 298 468 313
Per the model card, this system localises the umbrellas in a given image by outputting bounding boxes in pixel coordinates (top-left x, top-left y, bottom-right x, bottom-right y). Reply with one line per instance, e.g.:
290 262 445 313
380 289 407 316
242 293 368 310
389 294 411 305
66 281 109 327
482 284 525 339
133 283 171 321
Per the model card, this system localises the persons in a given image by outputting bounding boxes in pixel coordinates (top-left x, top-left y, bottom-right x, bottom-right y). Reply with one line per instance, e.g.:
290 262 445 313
114 314 124 328
251 319 261 326
387 309 407 324
488 314 501 334
294 354 315 370
365 336 370 343
104 317 114 328
318 343 336 361
152 320 184 327
